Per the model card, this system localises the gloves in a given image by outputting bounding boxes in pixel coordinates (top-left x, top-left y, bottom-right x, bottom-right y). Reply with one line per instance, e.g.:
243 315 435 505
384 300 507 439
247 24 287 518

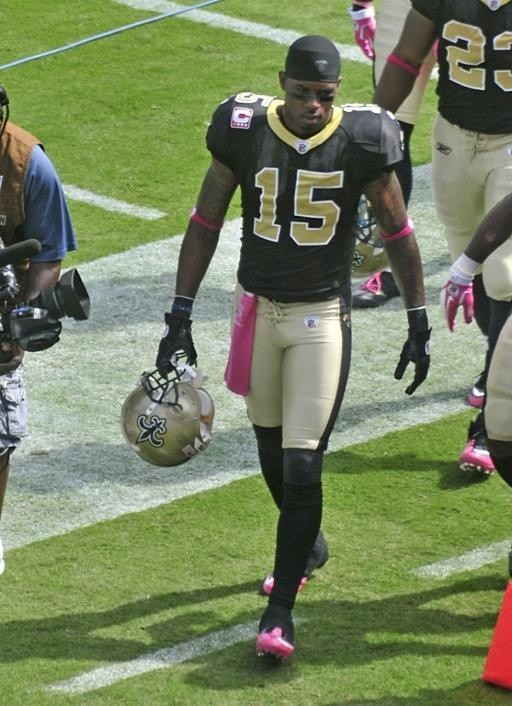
155 311 197 373
349 5 377 59
439 267 475 331
394 326 432 395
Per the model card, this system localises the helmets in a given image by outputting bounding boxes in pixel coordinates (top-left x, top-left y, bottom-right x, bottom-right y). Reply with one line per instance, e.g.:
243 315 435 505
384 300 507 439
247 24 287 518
349 199 392 278
120 352 214 466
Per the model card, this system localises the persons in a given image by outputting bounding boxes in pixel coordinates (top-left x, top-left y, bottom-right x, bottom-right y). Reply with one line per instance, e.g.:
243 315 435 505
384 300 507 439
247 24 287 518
155 34 433 664
370 1 512 476
443 193 512 580
0 85 78 575
348 0 440 309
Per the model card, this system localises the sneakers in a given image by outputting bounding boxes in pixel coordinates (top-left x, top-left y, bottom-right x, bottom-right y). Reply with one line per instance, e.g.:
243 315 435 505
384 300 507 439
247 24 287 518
258 609 296 663
459 412 496 474
263 532 328 596
467 369 488 406
351 269 401 306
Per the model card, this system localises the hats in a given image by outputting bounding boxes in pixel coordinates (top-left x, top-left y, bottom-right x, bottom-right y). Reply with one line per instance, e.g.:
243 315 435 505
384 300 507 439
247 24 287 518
285 34 341 82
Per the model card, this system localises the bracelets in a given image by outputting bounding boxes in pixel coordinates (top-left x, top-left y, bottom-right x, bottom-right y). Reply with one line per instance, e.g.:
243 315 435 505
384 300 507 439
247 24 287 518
449 252 481 280
